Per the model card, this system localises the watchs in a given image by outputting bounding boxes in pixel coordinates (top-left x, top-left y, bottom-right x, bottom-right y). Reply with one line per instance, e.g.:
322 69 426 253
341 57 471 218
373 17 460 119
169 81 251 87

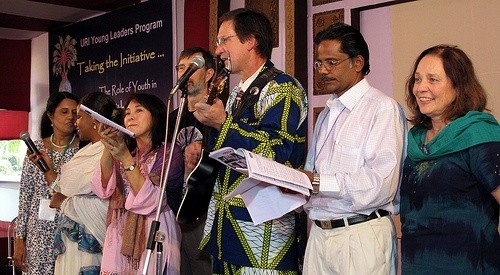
122 163 137 172
311 172 321 196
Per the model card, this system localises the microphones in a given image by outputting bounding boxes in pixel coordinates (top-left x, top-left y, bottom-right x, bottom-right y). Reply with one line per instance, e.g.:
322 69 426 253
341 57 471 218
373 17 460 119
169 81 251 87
20 131 50 173
170 56 205 95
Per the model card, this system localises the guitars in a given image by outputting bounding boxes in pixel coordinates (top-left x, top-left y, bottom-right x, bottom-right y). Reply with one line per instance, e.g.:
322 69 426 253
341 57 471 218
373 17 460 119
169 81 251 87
174 53 234 232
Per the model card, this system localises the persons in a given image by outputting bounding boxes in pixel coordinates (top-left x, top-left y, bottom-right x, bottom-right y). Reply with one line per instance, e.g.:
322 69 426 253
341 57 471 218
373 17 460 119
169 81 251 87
276 21 408 275
12 91 81 275
154 45 227 275
27 91 119 275
395 44 500 275
192 7 310 275
92 90 182 275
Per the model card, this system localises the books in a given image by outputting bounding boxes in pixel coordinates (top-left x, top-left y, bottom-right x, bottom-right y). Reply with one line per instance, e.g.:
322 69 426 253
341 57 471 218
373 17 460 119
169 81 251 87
80 102 137 139
209 147 313 196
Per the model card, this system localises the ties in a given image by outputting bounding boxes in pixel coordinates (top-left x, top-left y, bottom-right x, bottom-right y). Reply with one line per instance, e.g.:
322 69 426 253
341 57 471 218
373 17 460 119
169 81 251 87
236 90 244 107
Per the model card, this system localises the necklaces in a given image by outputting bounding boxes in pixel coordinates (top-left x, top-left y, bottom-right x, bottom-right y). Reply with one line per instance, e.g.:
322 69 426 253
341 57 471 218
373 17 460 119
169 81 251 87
50 132 76 149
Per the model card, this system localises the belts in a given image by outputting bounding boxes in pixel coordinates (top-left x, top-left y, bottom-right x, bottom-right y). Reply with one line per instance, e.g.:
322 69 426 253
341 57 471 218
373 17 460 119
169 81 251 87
315 208 392 229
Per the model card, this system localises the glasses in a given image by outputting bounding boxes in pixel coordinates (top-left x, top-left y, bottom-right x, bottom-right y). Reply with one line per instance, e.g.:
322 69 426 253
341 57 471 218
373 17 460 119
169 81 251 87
314 58 349 71
175 65 186 72
212 35 237 47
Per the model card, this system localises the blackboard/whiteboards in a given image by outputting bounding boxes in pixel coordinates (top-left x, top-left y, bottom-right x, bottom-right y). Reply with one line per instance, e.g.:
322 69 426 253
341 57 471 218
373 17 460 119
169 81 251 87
48 0 179 112
